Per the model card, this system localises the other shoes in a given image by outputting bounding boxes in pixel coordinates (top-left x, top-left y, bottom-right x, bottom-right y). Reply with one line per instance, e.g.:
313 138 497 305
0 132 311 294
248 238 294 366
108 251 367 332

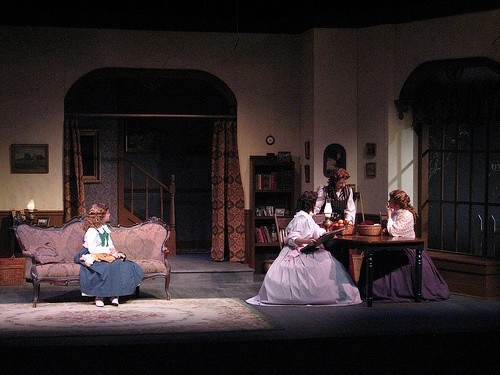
95 298 104 307
109 297 119 306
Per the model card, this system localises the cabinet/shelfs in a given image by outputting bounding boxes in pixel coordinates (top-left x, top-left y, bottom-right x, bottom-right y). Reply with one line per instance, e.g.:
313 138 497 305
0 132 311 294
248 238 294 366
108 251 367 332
249 152 295 269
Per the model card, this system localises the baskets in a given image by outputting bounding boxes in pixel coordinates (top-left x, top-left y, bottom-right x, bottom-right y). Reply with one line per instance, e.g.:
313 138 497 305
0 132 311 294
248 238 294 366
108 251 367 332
335 225 355 235
357 225 381 236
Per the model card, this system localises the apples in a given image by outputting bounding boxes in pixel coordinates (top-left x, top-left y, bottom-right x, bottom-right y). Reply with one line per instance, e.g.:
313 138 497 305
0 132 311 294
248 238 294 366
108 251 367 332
335 220 352 226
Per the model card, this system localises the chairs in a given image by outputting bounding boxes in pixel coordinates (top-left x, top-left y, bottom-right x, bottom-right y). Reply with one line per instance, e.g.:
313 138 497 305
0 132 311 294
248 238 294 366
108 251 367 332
272 214 327 256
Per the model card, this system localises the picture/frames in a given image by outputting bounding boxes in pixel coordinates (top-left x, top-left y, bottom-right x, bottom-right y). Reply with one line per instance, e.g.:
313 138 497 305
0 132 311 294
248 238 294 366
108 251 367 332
10 143 49 173
36 218 50 227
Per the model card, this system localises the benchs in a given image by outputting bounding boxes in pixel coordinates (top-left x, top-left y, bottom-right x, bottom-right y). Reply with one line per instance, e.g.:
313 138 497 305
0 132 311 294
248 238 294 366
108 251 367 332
16 215 171 308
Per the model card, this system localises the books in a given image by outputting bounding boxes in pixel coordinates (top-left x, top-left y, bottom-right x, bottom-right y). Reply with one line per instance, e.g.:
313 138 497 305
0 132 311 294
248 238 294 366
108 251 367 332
255 169 294 191
255 225 272 244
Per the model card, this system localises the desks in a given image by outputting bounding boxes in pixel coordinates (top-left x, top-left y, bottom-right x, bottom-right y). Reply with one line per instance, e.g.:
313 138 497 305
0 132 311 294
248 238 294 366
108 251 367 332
334 235 425 307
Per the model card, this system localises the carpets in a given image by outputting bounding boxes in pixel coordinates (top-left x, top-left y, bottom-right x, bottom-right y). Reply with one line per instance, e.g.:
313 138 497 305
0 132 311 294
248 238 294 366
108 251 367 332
0 297 285 339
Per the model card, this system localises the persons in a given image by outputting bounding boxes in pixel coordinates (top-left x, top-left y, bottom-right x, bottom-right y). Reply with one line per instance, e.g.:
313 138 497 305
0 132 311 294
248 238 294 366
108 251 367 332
74 202 146 307
308 168 357 260
368 189 451 302
245 190 363 307
